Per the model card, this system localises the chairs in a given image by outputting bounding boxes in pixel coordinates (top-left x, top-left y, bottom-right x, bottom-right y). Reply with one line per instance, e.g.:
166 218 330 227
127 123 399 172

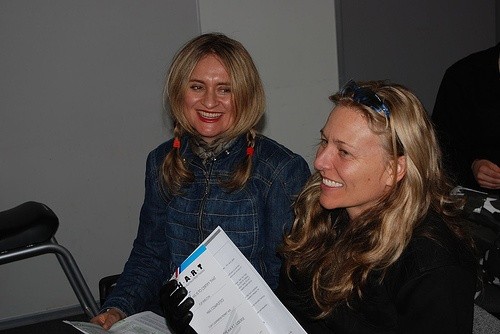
0 201 102 322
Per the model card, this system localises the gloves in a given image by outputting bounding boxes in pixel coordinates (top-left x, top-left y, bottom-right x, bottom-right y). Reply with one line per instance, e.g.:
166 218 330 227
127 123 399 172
158 276 197 334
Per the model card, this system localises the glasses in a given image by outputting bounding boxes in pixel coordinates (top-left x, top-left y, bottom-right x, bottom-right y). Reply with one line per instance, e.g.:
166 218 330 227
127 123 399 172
336 79 390 120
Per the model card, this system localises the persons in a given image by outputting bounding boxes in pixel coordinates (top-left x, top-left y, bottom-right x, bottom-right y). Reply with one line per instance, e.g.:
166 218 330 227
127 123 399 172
160 79 476 334
77 31 313 334
425 43 500 193
